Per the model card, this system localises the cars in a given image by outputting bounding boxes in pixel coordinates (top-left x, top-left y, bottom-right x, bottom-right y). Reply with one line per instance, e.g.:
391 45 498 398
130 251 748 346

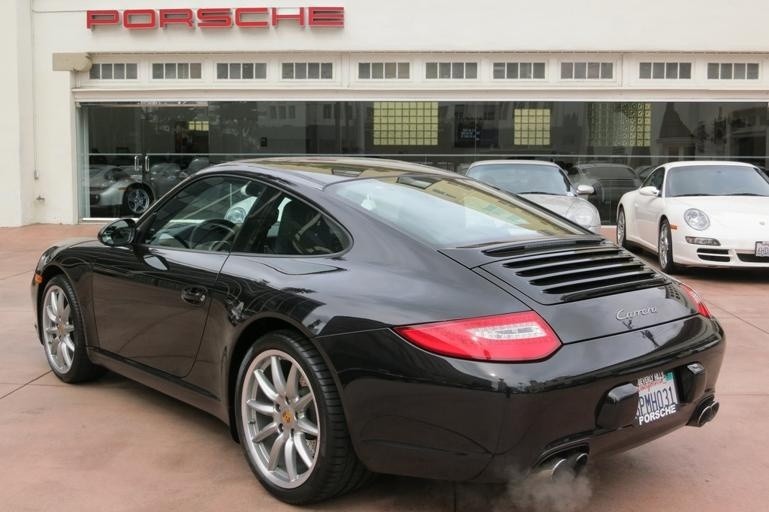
462 160 603 234
224 181 292 224
565 163 658 220
89 157 226 218
616 159 768 275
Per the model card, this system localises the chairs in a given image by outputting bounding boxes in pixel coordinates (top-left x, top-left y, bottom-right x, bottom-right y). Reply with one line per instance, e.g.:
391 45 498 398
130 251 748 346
275 200 342 256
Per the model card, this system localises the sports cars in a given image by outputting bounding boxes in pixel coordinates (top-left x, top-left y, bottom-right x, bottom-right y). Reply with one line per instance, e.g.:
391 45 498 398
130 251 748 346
30 154 727 507
